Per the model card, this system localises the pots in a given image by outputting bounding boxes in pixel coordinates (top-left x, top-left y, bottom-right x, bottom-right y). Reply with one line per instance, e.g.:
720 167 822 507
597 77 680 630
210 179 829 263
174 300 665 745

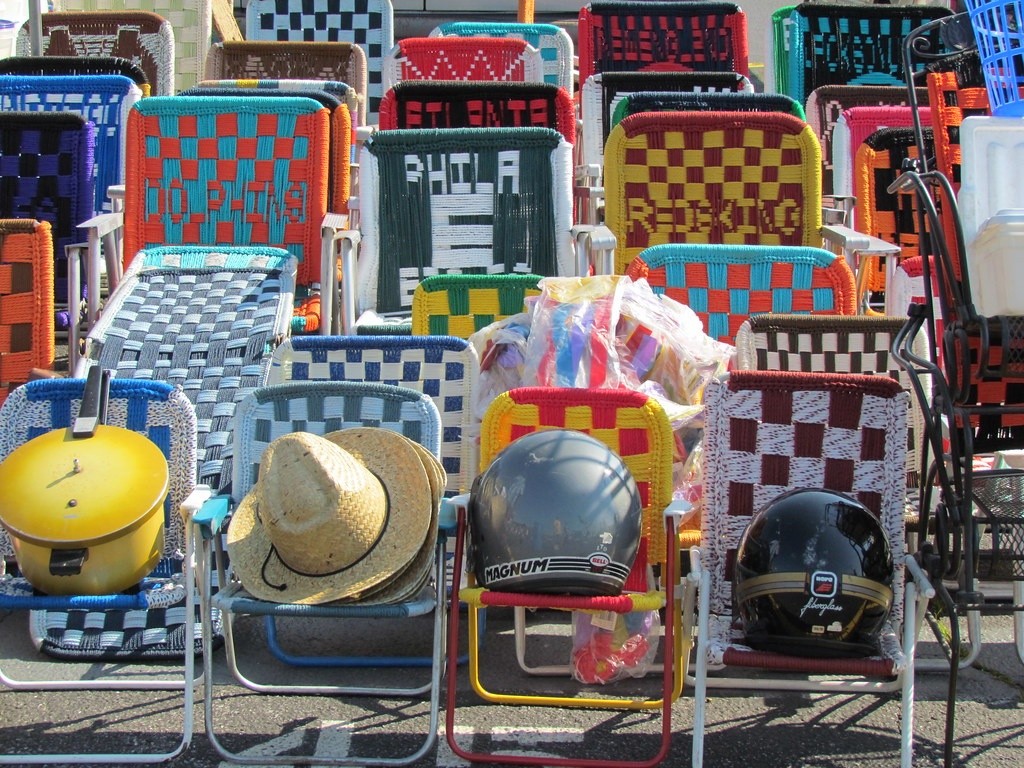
0 364 170 598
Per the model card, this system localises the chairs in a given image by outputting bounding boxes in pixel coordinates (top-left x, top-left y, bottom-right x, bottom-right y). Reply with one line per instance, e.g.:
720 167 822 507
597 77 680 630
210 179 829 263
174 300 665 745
0 0 1024 768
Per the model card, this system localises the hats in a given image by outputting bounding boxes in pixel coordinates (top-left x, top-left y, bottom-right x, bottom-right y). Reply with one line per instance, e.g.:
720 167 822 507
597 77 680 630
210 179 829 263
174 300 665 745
224 425 449 608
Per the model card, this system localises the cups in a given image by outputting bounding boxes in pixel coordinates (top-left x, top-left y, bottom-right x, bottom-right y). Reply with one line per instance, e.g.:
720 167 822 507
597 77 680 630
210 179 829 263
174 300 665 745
0 19 14 60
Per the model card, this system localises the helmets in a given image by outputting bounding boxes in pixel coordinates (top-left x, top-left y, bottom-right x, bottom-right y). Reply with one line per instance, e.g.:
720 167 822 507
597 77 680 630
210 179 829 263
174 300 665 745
467 426 643 602
725 487 895 656
